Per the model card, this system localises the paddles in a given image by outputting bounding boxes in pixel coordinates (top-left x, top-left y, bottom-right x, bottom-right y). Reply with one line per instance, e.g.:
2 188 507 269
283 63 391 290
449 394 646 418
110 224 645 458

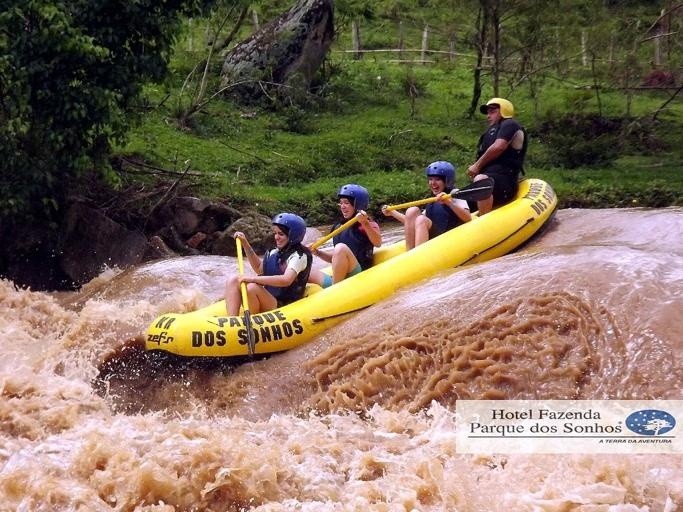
385 177 494 211
235 236 256 360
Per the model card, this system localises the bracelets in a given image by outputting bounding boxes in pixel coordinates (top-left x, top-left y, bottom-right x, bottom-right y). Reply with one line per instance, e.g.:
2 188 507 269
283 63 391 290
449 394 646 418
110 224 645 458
243 247 255 257
314 249 320 258
448 202 455 210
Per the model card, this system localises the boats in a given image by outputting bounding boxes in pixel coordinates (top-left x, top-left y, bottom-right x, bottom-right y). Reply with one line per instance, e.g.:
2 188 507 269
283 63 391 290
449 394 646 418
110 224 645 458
142 176 562 366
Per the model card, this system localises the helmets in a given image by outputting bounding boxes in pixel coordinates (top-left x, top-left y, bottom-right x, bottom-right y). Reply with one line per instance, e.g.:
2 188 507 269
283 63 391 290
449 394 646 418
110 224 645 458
485 98 513 119
337 183 369 210
271 213 306 246
426 160 455 188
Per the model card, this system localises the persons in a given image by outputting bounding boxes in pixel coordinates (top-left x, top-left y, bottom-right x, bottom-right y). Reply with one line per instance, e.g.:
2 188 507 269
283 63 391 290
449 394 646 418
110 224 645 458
223 212 312 315
307 184 381 287
450 97 528 216
381 161 472 250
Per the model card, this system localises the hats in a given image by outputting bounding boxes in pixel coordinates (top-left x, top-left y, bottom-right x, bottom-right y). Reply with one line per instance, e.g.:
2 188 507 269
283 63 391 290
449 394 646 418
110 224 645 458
479 104 498 114
